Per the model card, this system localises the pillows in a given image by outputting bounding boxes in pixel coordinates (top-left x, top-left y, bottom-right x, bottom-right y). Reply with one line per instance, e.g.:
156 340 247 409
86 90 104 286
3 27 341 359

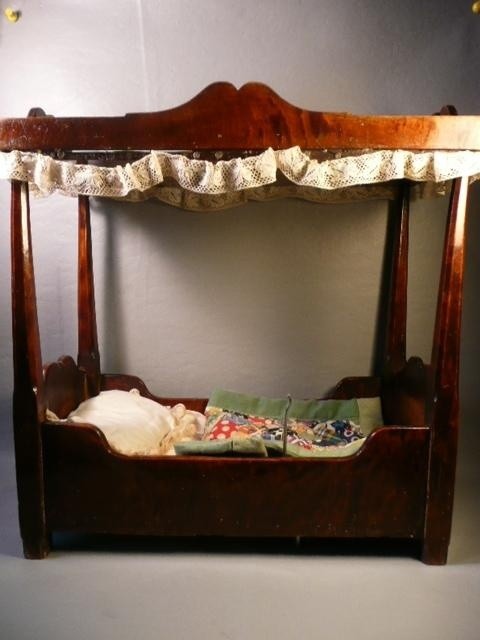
45 388 197 455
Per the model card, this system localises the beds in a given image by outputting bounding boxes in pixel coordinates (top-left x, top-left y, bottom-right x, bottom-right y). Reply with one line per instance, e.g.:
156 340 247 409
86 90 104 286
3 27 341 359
0 81 479 566
